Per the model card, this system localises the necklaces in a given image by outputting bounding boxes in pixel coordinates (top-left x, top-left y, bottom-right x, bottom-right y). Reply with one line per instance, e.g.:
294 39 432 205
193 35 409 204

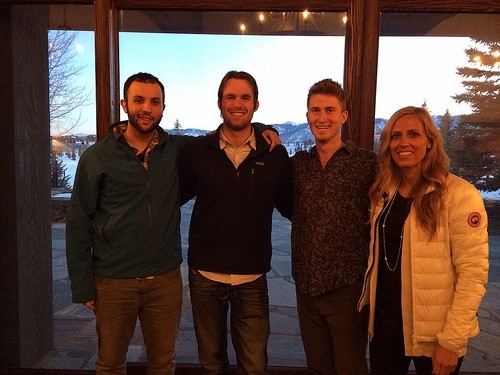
383 179 414 271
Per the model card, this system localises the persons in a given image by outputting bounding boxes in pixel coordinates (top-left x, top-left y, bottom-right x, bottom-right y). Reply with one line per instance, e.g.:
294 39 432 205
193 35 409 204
357 106 490 374
65 73 281 375
206 79 383 375
180 71 294 375
